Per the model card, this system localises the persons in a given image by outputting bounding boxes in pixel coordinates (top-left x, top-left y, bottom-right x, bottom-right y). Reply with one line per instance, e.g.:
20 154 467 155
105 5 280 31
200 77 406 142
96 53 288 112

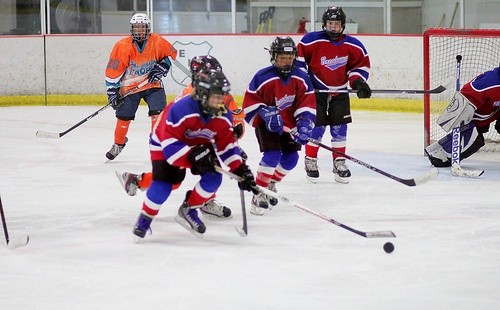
243 36 317 210
104 13 178 160
115 56 260 238
425 67 500 167
295 5 371 177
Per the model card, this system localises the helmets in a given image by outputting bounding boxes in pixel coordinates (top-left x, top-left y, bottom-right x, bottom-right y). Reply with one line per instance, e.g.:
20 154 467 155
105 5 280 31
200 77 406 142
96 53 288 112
322 6 346 40
190 54 232 119
129 13 153 41
264 35 298 83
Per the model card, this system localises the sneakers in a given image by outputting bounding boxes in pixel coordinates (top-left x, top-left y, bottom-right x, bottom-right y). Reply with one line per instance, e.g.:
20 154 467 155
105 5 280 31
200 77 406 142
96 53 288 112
265 181 279 210
249 192 269 215
105 136 128 163
199 198 231 220
332 157 351 184
115 168 145 196
133 212 153 243
303 155 319 183
175 189 206 240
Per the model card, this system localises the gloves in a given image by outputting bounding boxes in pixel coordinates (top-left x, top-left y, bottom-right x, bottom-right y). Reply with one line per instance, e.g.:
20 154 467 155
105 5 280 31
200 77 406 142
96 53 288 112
290 116 315 145
258 105 284 136
233 162 259 195
352 77 371 99
107 88 126 110
147 63 168 84
188 143 217 177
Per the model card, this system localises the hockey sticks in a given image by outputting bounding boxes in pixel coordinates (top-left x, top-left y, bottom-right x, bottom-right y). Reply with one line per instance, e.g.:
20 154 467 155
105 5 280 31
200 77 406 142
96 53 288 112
35 79 149 138
234 133 248 238
283 126 439 187
451 54 487 177
212 165 397 239
312 76 455 94
0 197 29 249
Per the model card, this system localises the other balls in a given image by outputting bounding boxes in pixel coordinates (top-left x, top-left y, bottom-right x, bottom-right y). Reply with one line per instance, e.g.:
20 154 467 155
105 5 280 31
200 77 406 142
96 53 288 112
383 241 394 253
